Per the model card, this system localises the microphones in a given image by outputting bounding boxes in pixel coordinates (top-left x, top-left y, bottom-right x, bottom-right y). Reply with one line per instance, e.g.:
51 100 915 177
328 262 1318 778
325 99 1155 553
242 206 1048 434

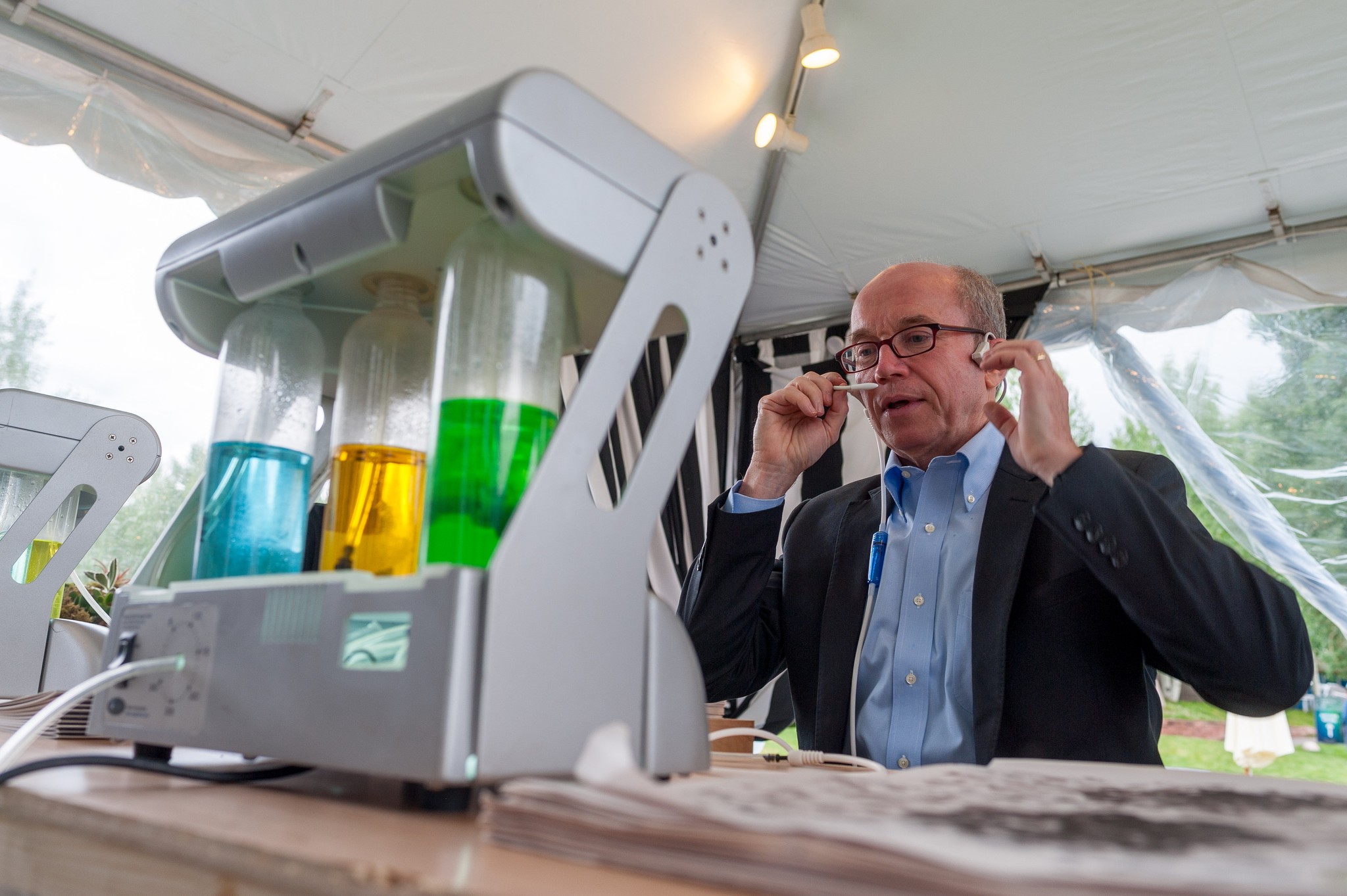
833 382 879 390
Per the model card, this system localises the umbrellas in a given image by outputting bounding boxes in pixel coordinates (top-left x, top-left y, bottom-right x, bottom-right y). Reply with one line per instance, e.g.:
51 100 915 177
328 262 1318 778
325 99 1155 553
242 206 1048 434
1224 710 1296 775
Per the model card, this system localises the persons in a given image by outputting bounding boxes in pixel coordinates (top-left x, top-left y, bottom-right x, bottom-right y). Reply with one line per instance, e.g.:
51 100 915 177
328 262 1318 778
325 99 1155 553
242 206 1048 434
678 263 1314 770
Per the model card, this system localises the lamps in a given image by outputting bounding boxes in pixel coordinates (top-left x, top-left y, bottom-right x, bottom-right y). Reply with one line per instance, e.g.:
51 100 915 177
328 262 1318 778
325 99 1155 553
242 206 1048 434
754 113 809 155
800 4 840 69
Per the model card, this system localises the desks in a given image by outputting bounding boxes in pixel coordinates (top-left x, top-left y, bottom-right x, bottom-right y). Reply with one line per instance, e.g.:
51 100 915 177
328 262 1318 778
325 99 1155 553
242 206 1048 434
0 712 1347 896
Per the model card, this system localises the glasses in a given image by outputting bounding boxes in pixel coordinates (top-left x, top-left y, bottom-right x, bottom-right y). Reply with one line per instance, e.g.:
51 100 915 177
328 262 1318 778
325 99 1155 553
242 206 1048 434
834 322 995 374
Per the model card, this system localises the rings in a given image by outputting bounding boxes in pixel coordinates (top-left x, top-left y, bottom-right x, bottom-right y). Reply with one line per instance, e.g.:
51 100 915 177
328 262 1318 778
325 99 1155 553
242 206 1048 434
1035 352 1046 363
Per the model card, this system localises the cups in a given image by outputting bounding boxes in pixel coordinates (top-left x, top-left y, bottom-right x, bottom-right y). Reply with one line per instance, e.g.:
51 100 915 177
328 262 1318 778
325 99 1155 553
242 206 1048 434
709 717 753 753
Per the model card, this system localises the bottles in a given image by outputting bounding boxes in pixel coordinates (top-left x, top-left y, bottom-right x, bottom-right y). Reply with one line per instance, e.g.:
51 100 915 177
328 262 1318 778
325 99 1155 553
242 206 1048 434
193 286 326 579
418 218 567 567
0 469 81 622
318 277 433 579
